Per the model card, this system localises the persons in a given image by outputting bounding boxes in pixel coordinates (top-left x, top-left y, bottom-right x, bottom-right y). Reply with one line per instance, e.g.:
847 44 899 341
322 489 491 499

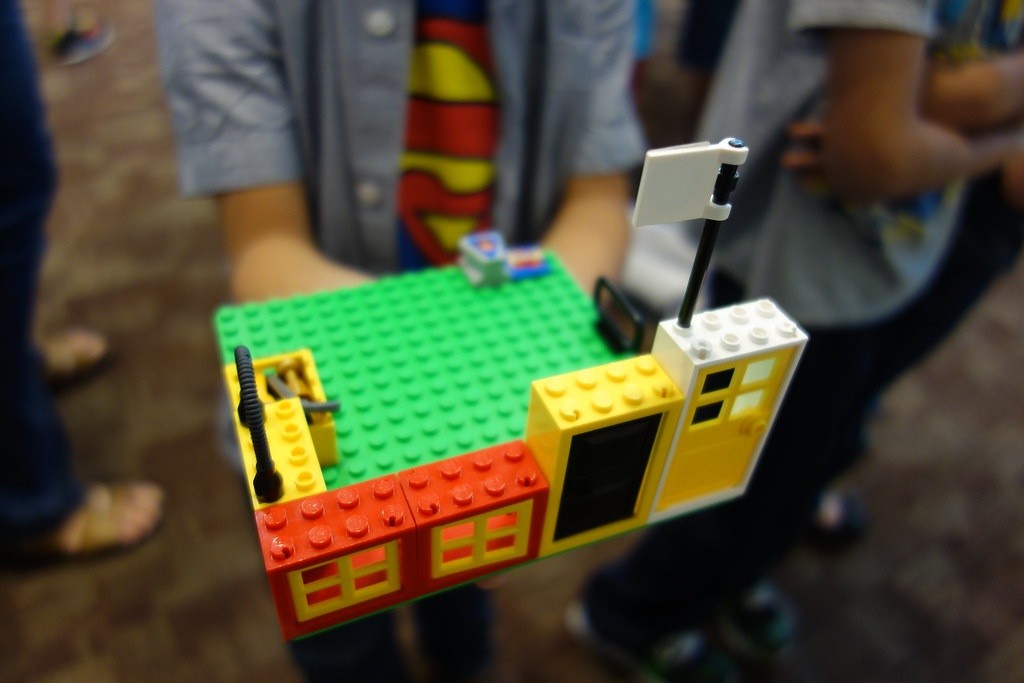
159 0 646 682
563 0 1024 683
0 0 166 572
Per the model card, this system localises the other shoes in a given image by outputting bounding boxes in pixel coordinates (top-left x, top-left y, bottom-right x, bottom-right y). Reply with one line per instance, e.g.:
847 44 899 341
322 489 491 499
44 327 113 390
54 23 115 66
717 581 800 665
807 492 865 553
44 481 167 561
565 581 740 683
